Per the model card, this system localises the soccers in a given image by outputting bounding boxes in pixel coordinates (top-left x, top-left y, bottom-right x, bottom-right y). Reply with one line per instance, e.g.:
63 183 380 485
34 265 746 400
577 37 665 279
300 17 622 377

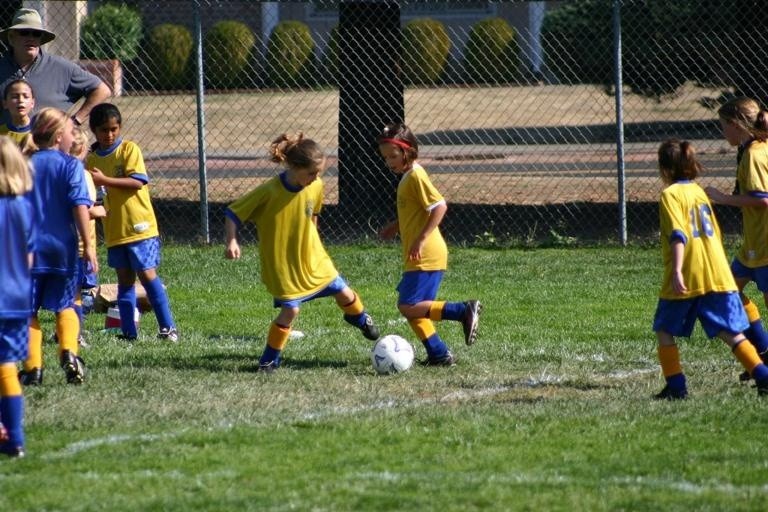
371 334 416 375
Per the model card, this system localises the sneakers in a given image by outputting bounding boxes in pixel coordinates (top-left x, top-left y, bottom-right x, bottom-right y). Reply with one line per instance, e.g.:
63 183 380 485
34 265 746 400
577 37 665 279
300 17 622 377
60 351 85 383
17 368 44 386
344 313 379 341
756 382 767 399
2 441 25 458
459 299 481 345
258 361 280 376
653 383 688 400
738 351 767 380
420 351 456 368
157 329 178 343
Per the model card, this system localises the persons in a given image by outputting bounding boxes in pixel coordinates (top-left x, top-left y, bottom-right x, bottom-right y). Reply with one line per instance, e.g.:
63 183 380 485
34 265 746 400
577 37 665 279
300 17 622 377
378 123 480 366
0 6 178 461
651 139 767 400
222 132 378 367
702 98 767 381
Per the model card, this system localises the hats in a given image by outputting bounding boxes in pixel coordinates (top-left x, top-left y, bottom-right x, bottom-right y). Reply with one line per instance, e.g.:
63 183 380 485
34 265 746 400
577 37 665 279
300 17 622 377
1 6 55 41
